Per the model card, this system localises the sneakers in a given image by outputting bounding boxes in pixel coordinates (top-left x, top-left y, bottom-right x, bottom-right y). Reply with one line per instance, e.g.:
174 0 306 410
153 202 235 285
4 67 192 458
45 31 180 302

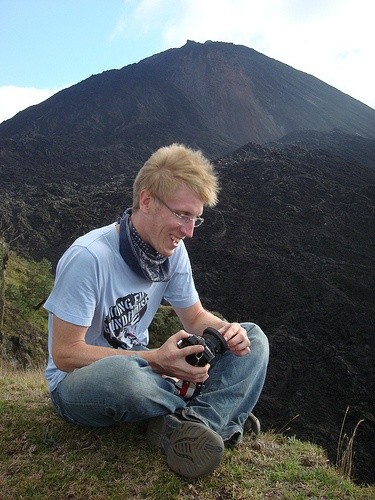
145 414 225 478
244 412 261 438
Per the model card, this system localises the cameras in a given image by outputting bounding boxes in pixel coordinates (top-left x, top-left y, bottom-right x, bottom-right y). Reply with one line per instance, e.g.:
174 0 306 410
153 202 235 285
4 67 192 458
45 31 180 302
176 327 227 373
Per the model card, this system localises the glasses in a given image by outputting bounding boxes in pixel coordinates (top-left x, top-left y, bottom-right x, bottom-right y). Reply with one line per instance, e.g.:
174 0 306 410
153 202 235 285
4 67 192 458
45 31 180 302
149 190 204 227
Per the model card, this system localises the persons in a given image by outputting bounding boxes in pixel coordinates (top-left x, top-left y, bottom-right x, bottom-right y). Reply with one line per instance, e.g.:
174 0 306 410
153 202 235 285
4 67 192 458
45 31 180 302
41 143 269 479
